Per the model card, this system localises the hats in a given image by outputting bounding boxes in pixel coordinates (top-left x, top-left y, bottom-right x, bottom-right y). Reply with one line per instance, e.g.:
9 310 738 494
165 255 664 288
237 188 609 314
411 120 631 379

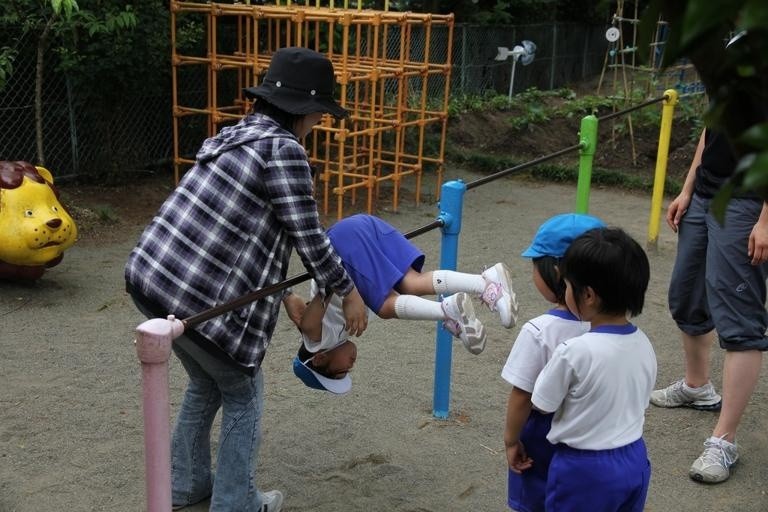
239 45 350 121
290 352 353 395
520 214 607 260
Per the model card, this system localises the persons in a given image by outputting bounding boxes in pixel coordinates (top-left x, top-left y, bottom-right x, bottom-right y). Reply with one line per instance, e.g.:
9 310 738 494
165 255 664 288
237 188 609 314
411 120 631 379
500 212 657 510
649 3 768 484
124 49 369 511
283 215 518 395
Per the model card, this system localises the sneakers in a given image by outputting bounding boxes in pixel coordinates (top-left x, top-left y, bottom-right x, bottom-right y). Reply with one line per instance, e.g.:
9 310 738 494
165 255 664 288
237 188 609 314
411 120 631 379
688 435 742 483
258 488 286 512
648 378 723 411
171 477 215 510
479 260 520 332
439 292 488 357
